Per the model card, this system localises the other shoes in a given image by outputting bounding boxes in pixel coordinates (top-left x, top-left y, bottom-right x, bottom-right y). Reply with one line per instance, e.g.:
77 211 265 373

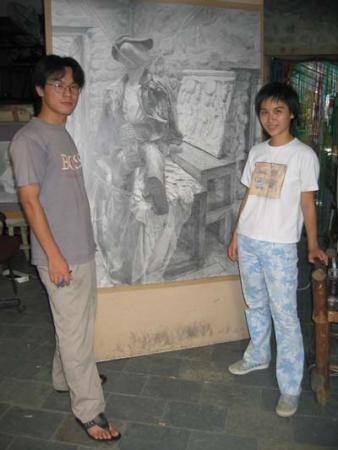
75 412 121 442
228 358 270 375
275 392 301 418
55 373 107 393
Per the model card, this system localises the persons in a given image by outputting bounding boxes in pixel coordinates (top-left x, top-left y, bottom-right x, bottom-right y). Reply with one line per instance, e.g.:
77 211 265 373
7 54 121 441
226 81 329 418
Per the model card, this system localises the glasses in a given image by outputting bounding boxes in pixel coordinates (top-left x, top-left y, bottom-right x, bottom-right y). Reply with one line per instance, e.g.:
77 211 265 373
45 81 82 96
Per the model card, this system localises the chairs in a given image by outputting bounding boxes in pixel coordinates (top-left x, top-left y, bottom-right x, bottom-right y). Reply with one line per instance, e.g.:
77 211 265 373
0 212 20 295
